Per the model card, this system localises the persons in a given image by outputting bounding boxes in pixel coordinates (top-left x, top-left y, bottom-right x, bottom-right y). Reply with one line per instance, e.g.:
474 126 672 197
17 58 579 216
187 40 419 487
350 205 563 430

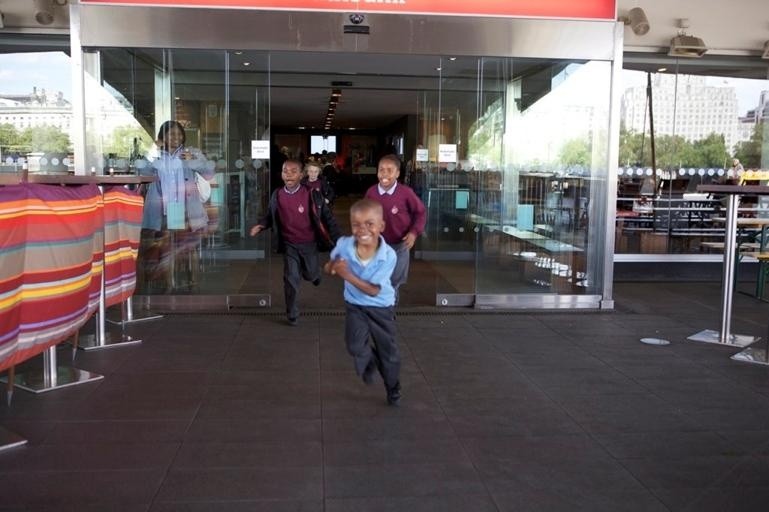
140 121 215 296
249 135 340 325
727 159 746 186
324 198 402 406
364 154 428 300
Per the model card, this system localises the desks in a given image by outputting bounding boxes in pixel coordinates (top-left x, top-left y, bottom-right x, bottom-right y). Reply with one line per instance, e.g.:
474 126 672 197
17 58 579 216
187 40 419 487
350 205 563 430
486 225 584 291
1 173 164 393
441 212 500 252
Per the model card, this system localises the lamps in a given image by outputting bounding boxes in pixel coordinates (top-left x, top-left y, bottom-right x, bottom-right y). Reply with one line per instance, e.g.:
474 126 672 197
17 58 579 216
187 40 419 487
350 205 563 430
324 89 342 130
762 40 769 59
667 19 708 57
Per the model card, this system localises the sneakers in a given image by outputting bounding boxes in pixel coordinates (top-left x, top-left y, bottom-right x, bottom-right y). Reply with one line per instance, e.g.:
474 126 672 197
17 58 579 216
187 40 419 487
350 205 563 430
385 380 401 405
363 345 378 385
313 276 322 286
288 317 297 325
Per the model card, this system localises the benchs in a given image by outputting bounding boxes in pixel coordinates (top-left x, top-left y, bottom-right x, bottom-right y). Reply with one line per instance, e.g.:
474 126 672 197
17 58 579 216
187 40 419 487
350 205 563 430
615 198 769 298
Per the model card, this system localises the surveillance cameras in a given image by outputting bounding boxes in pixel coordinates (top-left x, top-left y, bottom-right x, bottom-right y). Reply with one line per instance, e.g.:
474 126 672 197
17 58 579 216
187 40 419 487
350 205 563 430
628 8 650 35
